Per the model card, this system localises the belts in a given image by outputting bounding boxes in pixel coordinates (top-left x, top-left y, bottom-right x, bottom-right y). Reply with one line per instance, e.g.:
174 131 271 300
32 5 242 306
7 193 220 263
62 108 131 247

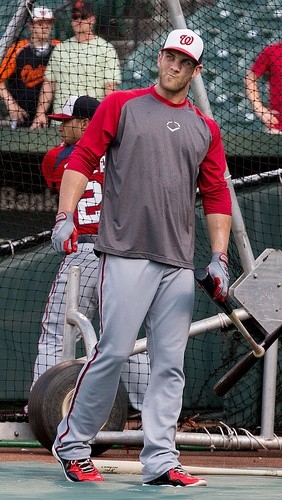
75 235 96 244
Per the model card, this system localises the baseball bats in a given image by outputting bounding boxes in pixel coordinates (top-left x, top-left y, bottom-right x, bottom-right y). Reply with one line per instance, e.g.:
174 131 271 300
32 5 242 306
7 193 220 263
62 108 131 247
213 324 282 398
193 267 266 358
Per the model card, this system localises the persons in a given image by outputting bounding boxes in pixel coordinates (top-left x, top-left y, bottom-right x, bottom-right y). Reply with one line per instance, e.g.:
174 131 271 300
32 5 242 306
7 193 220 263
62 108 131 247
30 0 123 130
244 39 282 135
24 96 151 414
0 6 63 127
51 28 233 487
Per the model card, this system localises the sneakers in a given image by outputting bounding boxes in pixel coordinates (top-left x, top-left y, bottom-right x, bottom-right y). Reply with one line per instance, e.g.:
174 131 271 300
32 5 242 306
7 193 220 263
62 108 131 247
52 444 104 482
143 464 207 487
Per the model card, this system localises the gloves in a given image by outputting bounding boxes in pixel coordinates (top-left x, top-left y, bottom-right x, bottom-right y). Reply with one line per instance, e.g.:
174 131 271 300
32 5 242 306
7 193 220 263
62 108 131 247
50 211 79 255
196 252 230 301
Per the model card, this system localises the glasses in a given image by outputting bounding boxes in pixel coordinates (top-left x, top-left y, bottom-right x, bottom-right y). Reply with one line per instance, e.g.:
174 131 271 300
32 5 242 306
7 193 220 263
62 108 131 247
72 14 91 20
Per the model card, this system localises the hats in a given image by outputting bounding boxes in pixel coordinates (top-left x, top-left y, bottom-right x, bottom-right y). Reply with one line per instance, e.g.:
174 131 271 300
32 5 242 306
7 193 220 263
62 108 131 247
161 28 205 65
70 0 94 15
47 95 100 120
31 6 55 21
